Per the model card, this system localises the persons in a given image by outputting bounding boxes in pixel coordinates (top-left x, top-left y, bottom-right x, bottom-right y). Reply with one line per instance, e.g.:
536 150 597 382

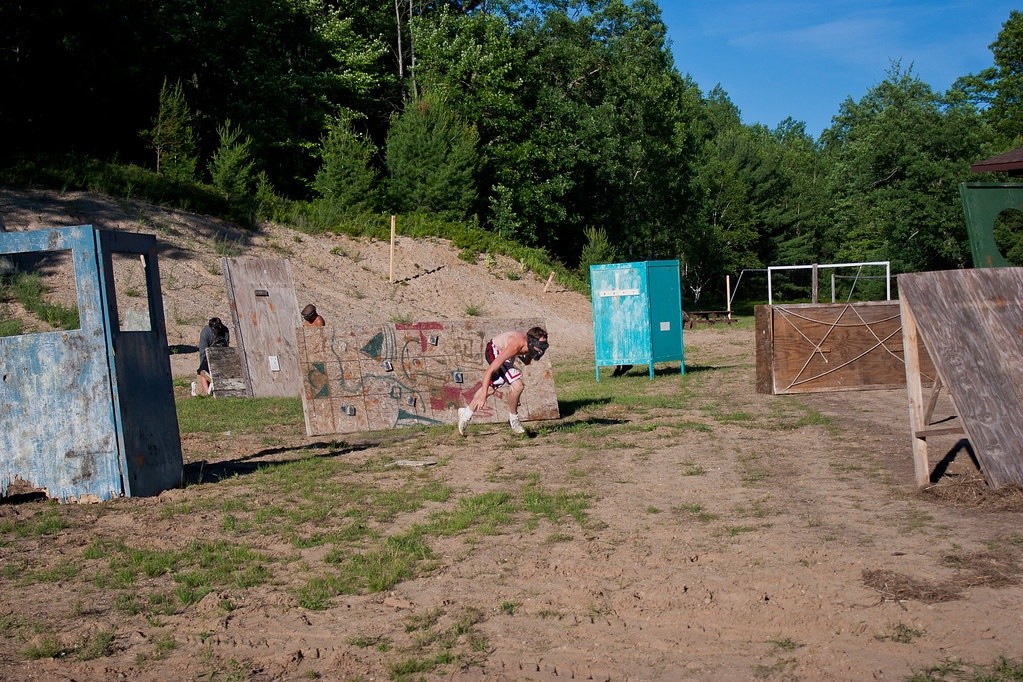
196 317 230 396
458 327 549 434
301 304 325 327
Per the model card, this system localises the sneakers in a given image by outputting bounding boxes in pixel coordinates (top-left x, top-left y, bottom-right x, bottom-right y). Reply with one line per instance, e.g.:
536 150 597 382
458 408 471 435
510 418 525 433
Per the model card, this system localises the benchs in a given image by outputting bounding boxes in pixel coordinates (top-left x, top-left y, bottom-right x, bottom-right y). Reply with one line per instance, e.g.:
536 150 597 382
687 318 739 324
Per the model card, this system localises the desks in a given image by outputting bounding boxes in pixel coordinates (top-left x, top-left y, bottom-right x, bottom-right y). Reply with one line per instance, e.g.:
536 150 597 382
687 311 735 329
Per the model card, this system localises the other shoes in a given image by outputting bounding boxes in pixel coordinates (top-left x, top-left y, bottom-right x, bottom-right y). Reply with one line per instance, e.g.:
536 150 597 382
191 382 197 396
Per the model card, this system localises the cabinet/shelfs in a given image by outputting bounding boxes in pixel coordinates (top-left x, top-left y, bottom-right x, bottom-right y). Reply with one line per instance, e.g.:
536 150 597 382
590 260 683 382
